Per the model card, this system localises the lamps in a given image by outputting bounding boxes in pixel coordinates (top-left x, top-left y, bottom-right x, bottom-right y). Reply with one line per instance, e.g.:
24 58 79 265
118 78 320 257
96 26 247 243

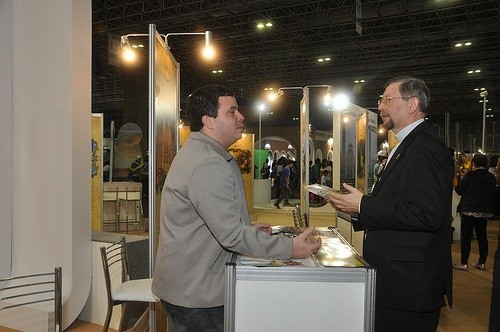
121 34 149 48
277 87 303 96
305 85 335 95
166 31 212 49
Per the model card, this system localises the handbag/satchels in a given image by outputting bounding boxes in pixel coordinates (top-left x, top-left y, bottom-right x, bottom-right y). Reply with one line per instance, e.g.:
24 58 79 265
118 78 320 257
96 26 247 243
260 168 266 176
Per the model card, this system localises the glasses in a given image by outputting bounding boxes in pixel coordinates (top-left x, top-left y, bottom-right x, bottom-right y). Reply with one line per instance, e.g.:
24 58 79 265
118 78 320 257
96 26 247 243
378 96 414 105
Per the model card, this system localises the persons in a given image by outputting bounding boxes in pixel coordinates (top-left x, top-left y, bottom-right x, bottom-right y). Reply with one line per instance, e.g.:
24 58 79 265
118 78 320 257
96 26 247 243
373 150 386 182
453 155 497 270
254 157 332 204
103 161 110 182
455 153 471 174
329 77 454 332
127 155 149 217
274 161 293 209
488 157 500 220
152 84 321 332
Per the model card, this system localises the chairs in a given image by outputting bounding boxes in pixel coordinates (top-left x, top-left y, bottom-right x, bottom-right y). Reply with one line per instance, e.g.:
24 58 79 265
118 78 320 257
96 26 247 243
100 235 160 332
0 266 62 332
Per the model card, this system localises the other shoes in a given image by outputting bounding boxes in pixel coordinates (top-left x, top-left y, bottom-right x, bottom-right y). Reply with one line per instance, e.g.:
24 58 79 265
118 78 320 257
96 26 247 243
284 202 291 205
274 203 281 209
453 263 468 271
472 263 486 270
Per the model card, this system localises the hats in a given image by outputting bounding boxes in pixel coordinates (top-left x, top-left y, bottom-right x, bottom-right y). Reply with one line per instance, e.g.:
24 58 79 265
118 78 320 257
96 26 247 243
378 150 387 156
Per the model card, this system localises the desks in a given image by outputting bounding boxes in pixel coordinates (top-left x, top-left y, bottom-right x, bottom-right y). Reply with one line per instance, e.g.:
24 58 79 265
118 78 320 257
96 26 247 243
224 225 377 332
